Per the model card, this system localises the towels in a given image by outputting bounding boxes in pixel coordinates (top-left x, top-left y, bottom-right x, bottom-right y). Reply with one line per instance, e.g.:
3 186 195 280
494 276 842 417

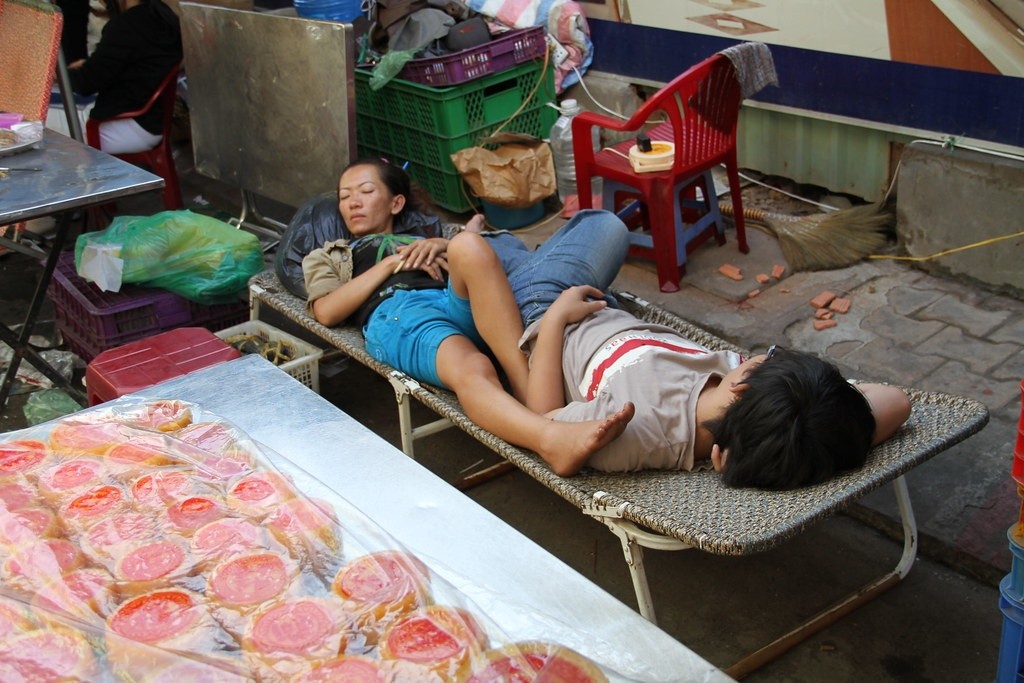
686 41 779 136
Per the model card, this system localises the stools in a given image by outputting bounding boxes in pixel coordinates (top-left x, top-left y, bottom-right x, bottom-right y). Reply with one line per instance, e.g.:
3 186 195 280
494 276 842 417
601 176 727 265
86 328 241 407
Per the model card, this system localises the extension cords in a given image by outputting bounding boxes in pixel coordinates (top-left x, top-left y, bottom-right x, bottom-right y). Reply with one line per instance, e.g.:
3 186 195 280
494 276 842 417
547 32 568 65
628 140 676 173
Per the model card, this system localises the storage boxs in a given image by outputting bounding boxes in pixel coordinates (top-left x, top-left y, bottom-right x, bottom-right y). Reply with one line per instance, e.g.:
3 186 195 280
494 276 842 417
41 256 224 367
213 319 324 395
354 49 559 214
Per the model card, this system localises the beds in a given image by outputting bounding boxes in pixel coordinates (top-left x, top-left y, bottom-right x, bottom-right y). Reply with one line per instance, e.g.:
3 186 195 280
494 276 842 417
247 222 992 682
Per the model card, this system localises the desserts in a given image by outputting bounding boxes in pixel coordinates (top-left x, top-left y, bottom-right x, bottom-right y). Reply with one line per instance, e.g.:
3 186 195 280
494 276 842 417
0 399 612 683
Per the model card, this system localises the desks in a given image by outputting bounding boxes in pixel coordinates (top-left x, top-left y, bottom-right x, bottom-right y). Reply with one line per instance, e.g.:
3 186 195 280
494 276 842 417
0 354 735 683
0 127 164 410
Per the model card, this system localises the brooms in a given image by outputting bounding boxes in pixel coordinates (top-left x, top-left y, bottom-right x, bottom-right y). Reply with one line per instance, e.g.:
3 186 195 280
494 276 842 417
718 188 894 273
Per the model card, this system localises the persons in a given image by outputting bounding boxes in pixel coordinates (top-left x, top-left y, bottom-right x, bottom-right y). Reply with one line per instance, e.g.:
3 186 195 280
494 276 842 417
67 0 183 154
303 158 635 478
467 209 911 493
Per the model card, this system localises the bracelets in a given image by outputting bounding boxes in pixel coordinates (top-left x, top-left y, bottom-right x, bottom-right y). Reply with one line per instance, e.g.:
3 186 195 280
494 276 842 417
393 256 407 274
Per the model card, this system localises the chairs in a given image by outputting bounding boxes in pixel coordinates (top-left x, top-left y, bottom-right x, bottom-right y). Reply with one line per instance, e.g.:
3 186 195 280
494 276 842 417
569 43 779 293
86 61 182 211
